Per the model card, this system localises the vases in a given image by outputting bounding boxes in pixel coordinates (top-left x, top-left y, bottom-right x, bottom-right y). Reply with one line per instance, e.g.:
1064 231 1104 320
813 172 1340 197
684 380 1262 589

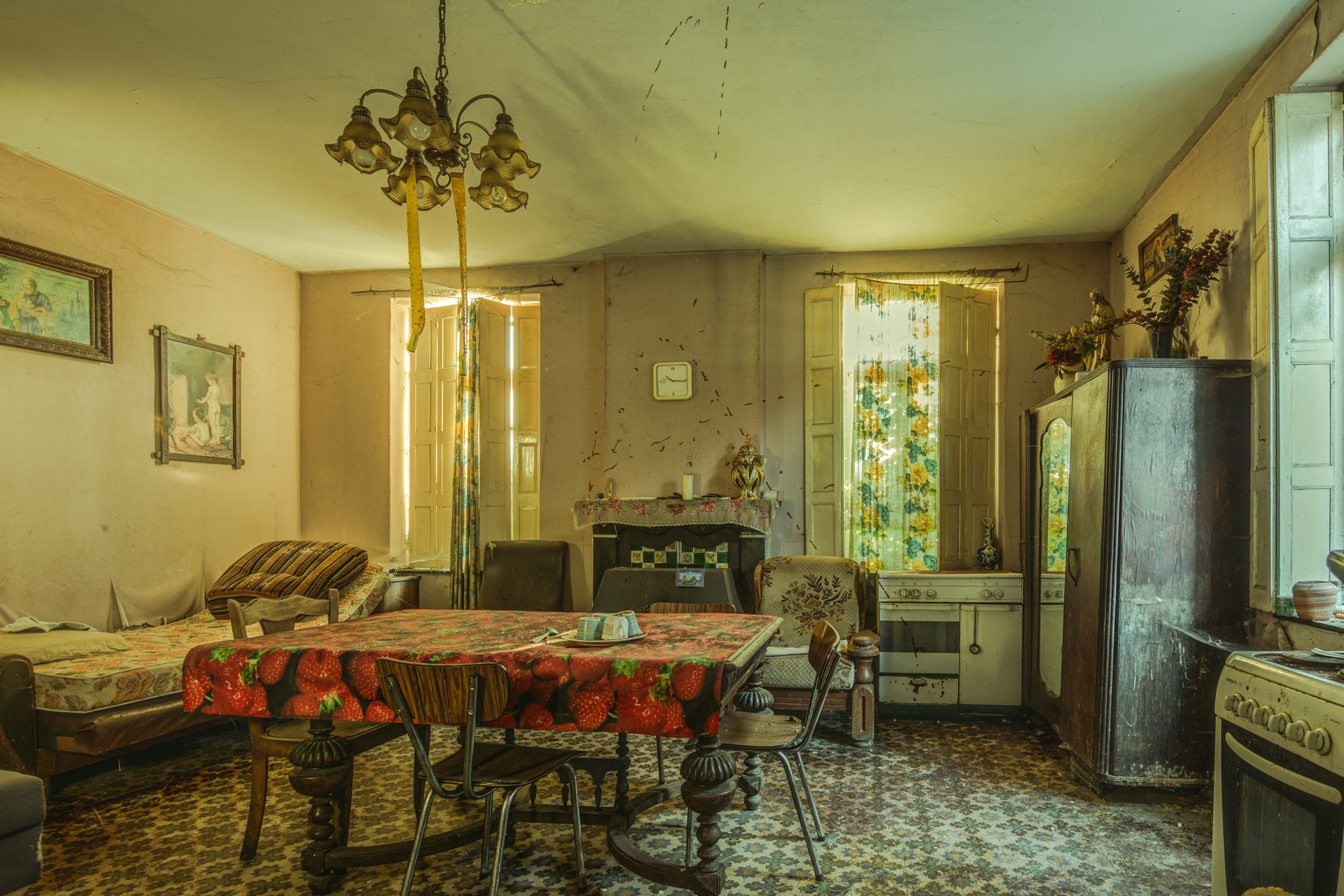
1143 321 1176 359
975 514 1000 570
1054 362 1084 393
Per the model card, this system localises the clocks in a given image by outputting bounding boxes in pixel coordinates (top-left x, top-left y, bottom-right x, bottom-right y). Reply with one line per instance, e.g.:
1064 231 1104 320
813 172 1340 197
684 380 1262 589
652 361 693 401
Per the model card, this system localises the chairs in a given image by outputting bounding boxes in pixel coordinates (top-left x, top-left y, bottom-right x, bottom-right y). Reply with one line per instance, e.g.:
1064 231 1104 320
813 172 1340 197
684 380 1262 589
675 610 847 882
476 535 570 611
221 588 430 865
374 655 592 896
752 552 878 750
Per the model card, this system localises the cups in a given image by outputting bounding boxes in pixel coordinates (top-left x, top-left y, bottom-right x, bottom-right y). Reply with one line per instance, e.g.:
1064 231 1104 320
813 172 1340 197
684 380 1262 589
591 614 613 637
576 617 601 640
601 615 628 639
614 610 640 636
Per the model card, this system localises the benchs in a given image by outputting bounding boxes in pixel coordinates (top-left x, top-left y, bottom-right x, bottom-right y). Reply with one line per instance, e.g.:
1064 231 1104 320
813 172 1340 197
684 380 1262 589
0 534 398 801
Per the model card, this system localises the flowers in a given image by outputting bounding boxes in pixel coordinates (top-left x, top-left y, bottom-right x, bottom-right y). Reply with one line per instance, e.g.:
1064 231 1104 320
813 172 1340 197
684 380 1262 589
1028 217 1239 374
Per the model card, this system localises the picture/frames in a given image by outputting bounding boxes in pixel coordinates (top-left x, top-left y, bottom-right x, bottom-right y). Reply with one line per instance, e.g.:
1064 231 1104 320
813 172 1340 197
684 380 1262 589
1137 213 1179 292
0 235 114 363
149 324 246 471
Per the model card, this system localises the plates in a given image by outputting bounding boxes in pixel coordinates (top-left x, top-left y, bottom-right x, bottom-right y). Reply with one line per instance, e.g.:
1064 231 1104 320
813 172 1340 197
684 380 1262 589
561 630 648 646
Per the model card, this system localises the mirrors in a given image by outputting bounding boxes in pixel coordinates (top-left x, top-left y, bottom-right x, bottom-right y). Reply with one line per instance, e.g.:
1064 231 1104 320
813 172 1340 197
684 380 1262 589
1034 396 1074 703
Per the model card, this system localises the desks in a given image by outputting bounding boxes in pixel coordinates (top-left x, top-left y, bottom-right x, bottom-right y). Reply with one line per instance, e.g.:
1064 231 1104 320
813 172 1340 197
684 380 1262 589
573 496 776 612
182 600 784 896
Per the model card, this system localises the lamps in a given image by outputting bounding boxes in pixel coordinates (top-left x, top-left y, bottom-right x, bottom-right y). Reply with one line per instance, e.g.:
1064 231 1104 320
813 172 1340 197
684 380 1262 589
322 0 546 213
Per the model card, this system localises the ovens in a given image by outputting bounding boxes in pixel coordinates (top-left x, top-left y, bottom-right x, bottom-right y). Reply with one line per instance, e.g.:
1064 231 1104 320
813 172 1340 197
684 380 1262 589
1211 715 1344 896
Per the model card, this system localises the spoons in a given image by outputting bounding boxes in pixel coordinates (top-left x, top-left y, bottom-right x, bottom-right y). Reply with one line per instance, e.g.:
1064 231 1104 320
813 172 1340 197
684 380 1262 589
532 627 559 643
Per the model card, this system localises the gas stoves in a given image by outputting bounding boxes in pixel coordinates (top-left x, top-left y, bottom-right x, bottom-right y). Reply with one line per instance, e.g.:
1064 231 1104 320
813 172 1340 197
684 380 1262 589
1215 650 1344 776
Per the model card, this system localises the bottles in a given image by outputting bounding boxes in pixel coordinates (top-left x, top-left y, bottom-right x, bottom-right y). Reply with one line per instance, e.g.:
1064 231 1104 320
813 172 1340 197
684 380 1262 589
682 464 694 500
389 556 398 570
976 516 1001 570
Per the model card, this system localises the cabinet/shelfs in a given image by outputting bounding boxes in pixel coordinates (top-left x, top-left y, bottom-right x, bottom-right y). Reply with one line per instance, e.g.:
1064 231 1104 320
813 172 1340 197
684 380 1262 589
1007 353 1255 800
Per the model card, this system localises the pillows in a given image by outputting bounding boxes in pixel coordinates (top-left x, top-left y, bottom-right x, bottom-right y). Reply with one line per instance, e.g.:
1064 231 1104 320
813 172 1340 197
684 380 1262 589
205 538 370 620
